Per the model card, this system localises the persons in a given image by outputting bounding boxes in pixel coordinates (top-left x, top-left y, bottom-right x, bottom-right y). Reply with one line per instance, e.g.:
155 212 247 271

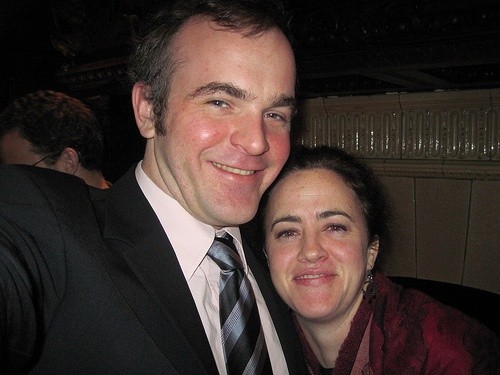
0 88 113 190
239 144 500 375
0 0 306 375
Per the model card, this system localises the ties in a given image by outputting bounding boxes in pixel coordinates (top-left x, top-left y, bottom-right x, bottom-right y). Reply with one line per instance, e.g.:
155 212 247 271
206 232 274 375
